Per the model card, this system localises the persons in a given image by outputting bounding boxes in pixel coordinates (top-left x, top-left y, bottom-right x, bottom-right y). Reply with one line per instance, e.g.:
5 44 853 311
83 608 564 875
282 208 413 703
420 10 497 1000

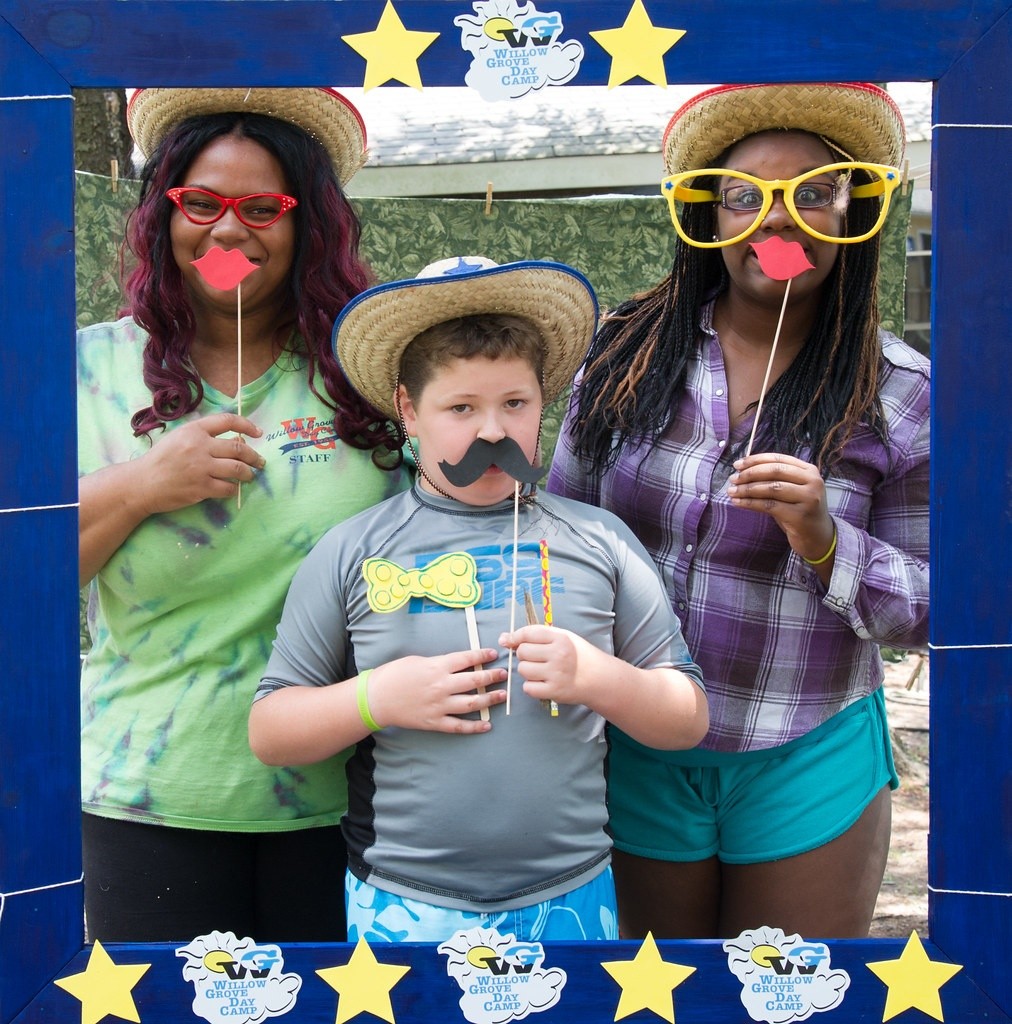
249 254 710 944
66 87 420 943
546 81 929 939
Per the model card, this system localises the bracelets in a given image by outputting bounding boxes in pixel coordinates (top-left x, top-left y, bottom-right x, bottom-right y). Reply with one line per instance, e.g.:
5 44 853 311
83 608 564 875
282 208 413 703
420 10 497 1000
358 668 384 732
802 527 837 564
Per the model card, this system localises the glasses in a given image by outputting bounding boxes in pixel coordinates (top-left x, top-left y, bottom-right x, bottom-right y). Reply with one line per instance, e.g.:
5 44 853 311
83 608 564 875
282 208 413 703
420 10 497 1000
164 186 299 229
661 162 903 249
720 181 836 210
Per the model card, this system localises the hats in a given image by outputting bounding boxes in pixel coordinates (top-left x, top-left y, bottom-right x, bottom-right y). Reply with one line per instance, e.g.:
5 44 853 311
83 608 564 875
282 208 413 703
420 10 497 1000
661 82 906 191
331 256 600 423
127 88 368 189
